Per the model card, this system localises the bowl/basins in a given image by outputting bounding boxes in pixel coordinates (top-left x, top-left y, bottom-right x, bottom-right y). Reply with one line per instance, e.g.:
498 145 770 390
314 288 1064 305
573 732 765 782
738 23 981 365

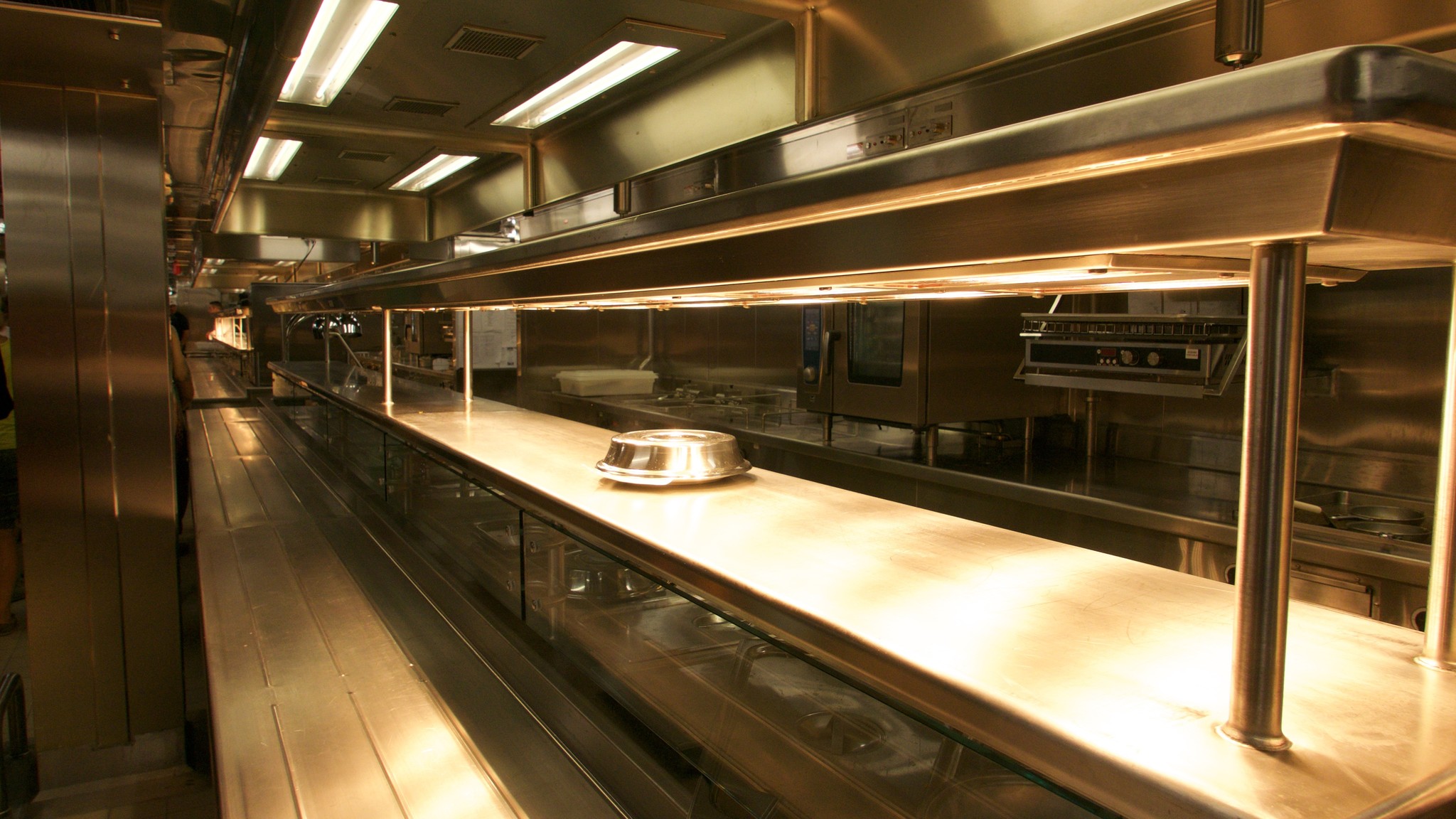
595 428 752 478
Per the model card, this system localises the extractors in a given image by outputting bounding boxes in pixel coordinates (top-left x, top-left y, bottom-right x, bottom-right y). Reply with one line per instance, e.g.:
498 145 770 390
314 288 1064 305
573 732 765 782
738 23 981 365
1025 338 1244 378
